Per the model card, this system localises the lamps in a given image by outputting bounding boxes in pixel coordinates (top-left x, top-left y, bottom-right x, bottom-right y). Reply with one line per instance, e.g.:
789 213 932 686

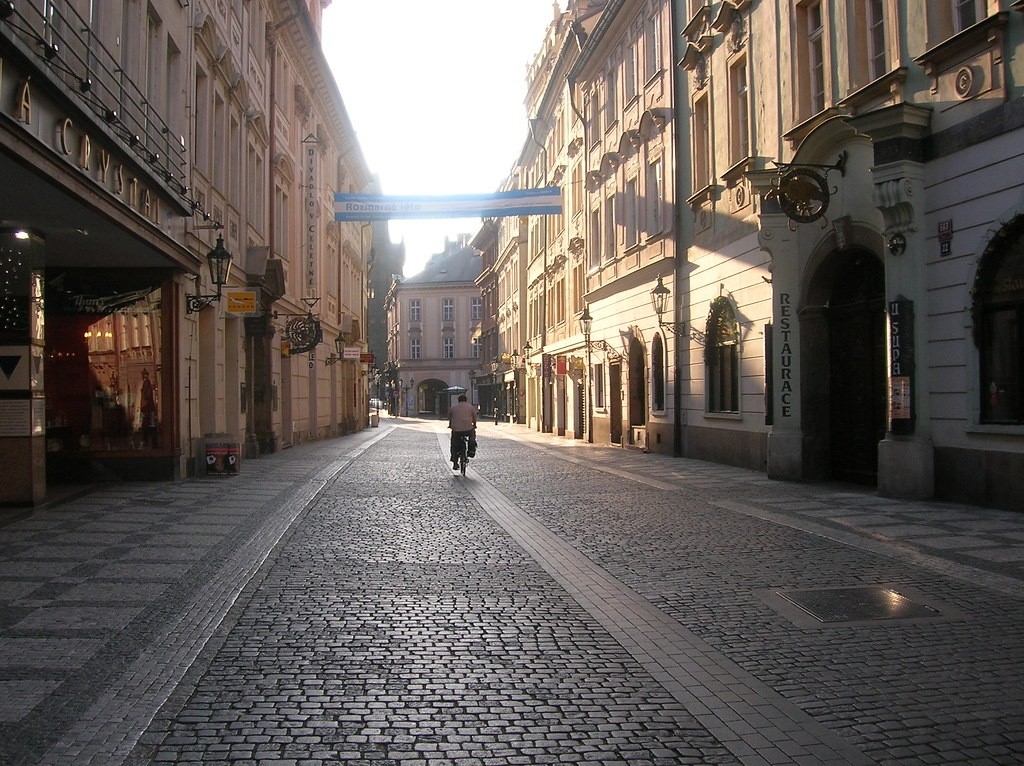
407 378 414 391
650 272 686 338
361 364 379 376
577 305 606 352
185 232 234 314
325 332 346 367
491 355 510 373
511 349 527 374
522 341 542 370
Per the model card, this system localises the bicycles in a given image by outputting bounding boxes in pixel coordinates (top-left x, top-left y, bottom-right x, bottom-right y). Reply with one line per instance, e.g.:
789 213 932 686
447 425 477 476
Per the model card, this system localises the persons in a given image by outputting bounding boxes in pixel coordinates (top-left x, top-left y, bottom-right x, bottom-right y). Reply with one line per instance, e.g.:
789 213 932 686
449 395 477 470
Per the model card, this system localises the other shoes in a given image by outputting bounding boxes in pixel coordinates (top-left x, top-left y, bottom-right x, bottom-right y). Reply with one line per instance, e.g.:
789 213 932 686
467 451 475 457
453 464 459 469
